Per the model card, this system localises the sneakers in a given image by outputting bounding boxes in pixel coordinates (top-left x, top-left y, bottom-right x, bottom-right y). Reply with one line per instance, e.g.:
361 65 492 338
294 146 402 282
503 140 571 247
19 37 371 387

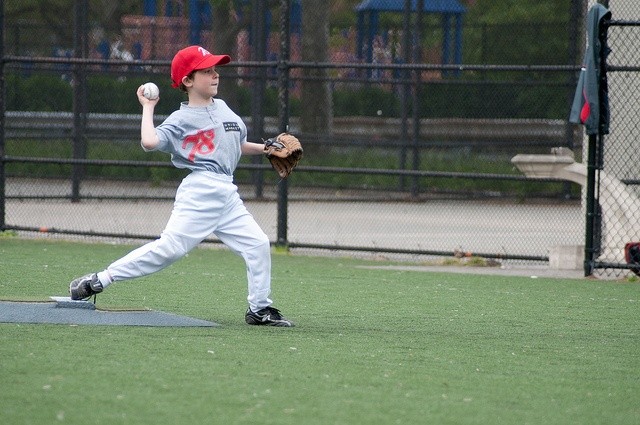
245 306 295 327
70 272 103 304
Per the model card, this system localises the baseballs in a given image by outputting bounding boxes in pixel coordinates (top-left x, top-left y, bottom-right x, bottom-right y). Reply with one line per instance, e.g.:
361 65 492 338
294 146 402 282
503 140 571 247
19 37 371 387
143 82 159 99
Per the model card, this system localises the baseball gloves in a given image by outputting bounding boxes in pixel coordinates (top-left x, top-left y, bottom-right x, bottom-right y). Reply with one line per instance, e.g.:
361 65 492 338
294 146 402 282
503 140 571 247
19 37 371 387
261 133 303 178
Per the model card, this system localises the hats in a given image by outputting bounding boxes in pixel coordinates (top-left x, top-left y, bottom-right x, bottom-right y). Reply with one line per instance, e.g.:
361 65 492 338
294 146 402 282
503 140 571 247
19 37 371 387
171 45 232 88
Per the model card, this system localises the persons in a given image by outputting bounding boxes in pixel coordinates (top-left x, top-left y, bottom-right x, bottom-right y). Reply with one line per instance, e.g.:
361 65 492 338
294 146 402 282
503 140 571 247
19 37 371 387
69 46 295 328
